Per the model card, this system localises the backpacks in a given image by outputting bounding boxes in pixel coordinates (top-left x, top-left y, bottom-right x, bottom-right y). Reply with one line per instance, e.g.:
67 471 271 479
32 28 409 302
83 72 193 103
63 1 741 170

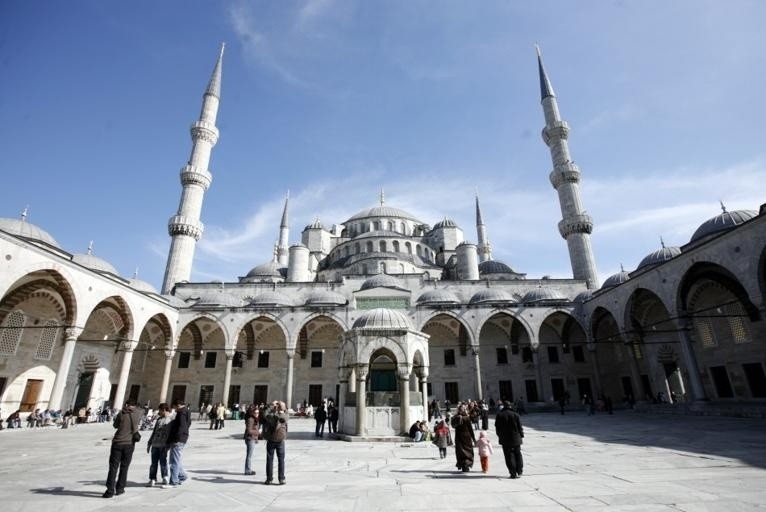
269 415 288 444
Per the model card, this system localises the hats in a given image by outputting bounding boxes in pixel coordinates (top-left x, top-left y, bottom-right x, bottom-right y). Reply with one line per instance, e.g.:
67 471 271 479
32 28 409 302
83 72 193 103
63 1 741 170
503 400 512 407
479 430 487 438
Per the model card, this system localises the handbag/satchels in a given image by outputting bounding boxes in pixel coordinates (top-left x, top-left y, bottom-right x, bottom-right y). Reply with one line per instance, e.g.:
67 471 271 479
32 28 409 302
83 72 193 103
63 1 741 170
131 430 141 442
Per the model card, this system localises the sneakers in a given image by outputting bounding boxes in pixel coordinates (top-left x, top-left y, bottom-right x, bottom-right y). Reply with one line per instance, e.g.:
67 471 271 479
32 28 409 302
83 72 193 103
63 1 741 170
115 488 125 495
244 470 256 475
102 489 114 498
145 475 190 489
264 478 274 485
278 477 288 485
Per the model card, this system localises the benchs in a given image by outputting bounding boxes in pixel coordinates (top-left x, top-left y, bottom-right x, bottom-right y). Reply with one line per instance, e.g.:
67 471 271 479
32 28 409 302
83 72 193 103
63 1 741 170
1 410 107 428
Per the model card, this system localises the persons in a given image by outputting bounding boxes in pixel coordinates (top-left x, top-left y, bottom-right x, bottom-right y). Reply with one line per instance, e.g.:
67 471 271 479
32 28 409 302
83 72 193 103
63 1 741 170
160 399 191 489
263 400 289 484
244 408 260 475
495 400 524 479
558 390 677 417
0 395 339 438
409 396 528 445
474 431 494 474
451 405 476 472
432 420 453 459
102 400 143 498
145 403 175 486
